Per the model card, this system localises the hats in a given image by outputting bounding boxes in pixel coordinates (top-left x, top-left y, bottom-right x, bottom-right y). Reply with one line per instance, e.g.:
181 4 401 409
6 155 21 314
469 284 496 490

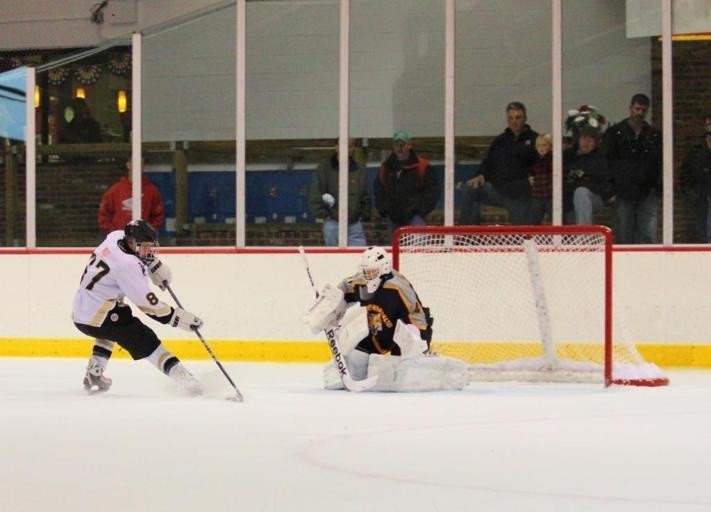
393 131 408 144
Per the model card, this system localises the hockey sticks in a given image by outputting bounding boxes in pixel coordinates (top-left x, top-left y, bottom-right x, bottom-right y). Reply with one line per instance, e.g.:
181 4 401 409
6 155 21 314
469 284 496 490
296 244 378 392
162 281 243 402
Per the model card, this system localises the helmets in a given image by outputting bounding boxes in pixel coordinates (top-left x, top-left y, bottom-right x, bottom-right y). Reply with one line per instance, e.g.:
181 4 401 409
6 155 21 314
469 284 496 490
124 219 159 260
361 246 391 294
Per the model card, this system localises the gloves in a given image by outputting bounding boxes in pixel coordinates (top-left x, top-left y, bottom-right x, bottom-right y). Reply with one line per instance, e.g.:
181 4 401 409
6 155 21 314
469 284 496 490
169 308 203 332
148 259 171 290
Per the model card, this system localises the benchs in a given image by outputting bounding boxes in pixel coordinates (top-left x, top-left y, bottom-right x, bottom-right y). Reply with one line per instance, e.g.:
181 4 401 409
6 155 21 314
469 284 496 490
172 190 699 247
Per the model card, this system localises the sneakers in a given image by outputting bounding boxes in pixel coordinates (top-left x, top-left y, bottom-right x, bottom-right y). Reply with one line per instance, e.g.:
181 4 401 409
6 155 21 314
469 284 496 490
84 372 112 390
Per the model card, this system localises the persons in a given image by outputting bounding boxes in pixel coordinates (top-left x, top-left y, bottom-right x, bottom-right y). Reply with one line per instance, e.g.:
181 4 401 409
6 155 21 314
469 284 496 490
681 132 711 242
97 158 164 234
374 131 441 245
562 125 608 225
310 137 372 246
57 99 100 161
529 133 552 225
74 218 201 391
305 247 433 391
459 102 538 225
604 93 661 244
